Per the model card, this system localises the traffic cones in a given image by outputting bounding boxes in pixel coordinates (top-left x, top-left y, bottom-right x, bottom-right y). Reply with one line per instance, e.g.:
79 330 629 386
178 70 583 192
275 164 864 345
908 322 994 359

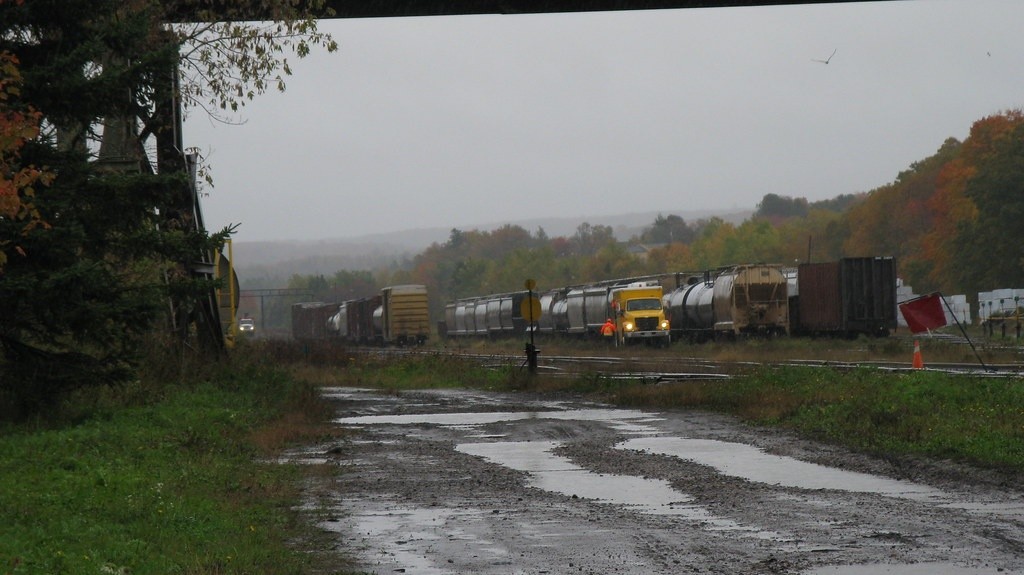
912 341 923 369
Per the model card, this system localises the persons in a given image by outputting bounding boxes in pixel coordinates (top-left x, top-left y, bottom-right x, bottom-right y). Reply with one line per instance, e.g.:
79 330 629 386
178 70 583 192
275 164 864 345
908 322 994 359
600 318 616 344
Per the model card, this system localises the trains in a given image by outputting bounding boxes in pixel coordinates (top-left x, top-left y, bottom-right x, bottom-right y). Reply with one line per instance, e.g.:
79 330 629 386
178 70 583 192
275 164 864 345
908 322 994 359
437 256 900 343
291 285 430 348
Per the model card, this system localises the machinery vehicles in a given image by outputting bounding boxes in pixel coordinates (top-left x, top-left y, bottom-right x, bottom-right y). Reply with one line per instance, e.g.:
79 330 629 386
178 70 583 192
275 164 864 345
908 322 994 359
611 286 671 347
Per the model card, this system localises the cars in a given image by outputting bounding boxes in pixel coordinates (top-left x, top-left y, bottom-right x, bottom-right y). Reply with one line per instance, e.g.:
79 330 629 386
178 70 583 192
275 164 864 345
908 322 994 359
239 319 254 335
